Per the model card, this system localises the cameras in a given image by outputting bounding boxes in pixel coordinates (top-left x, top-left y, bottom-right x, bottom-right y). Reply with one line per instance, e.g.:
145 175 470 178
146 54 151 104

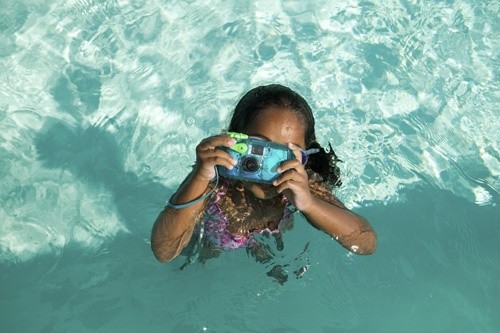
216 134 295 186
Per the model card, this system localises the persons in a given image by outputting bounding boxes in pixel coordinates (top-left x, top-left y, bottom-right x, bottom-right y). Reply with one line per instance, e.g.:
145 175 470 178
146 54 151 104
150 85 376 265
